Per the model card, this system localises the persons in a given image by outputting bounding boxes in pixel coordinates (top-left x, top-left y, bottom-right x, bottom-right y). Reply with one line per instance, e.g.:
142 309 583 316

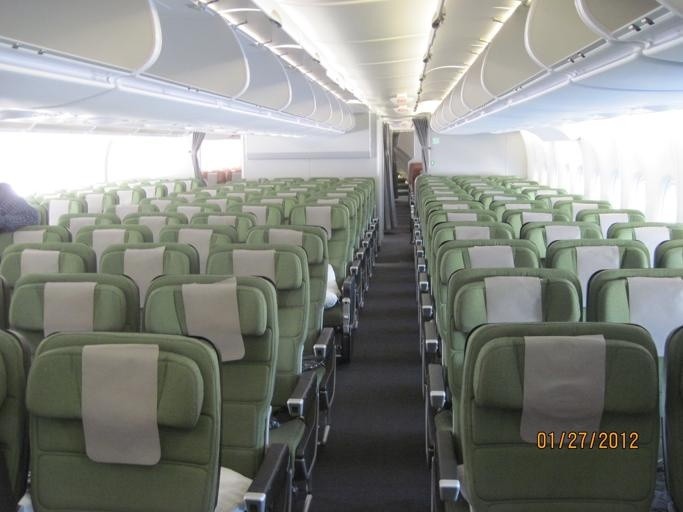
0 182 41 231
